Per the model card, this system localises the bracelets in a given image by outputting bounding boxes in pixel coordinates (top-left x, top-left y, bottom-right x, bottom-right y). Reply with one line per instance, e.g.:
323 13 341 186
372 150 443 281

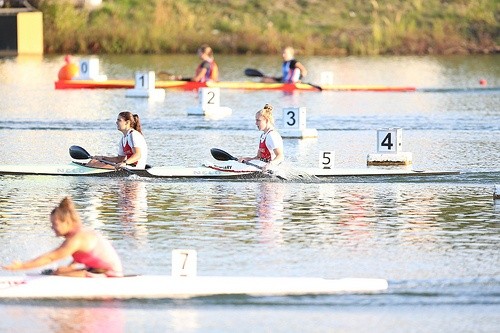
299 74 303 80
123 160 127 165
102 156 105 160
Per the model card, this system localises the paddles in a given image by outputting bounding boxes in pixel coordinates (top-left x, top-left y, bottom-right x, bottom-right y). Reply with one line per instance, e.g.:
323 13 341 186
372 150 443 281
157 70 191 81
211 148 288 182
244 68 323 91
69 145 152 177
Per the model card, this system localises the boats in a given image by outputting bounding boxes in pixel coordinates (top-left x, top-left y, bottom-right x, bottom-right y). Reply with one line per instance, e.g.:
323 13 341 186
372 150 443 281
1 265 388 301
0 147 461 182
53 57 415 97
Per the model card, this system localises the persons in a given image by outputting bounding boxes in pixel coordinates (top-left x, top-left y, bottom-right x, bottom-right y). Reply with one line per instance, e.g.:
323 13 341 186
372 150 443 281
2 196 123 278
261 45 307 84
89 112 148 170
239 104 284 172
169 43 220 83
